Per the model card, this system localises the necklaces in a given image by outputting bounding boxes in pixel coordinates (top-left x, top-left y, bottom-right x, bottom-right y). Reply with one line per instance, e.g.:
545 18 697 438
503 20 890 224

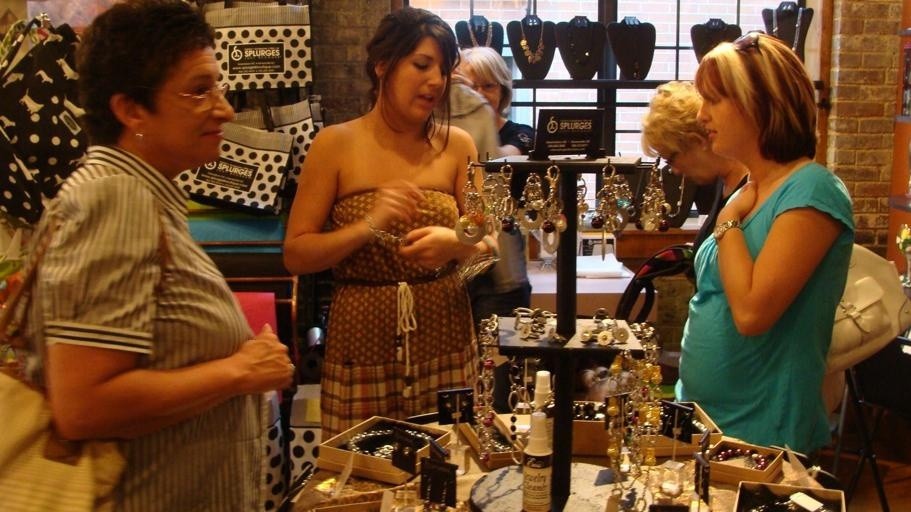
606 349 664 512
521 21 545 64
467 21 493 46
348 429 432 459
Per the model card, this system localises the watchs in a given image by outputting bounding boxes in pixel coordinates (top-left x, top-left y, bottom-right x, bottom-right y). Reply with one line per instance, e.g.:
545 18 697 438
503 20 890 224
714 220 742 239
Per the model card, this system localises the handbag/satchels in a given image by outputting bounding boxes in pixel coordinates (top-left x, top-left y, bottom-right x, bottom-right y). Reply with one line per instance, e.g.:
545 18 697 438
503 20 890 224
824 243 911 375
0 210 170 511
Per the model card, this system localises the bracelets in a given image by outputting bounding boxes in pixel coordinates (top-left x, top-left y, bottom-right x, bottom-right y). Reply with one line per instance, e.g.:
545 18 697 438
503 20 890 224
715 447 767 470
484 235 497 271
364 214 401 244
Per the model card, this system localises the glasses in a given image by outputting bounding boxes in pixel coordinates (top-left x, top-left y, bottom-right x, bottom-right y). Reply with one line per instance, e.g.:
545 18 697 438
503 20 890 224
472 81 499 90
173 85 228 114
734 30 764 54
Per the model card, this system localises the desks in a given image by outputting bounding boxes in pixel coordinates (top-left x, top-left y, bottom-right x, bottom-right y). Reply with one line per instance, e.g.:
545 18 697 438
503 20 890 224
289 408 846 510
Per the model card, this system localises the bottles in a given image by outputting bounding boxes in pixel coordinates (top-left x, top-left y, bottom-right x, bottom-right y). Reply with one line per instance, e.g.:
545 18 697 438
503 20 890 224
533 370 552 409
522 411 552 511
900 29 911 117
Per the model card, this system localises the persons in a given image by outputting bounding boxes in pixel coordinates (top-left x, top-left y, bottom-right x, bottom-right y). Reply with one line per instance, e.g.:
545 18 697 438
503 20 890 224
455 46 550 413
283 7 500 447
27 40 296 512
644 80 748 280
674 31 856 467
